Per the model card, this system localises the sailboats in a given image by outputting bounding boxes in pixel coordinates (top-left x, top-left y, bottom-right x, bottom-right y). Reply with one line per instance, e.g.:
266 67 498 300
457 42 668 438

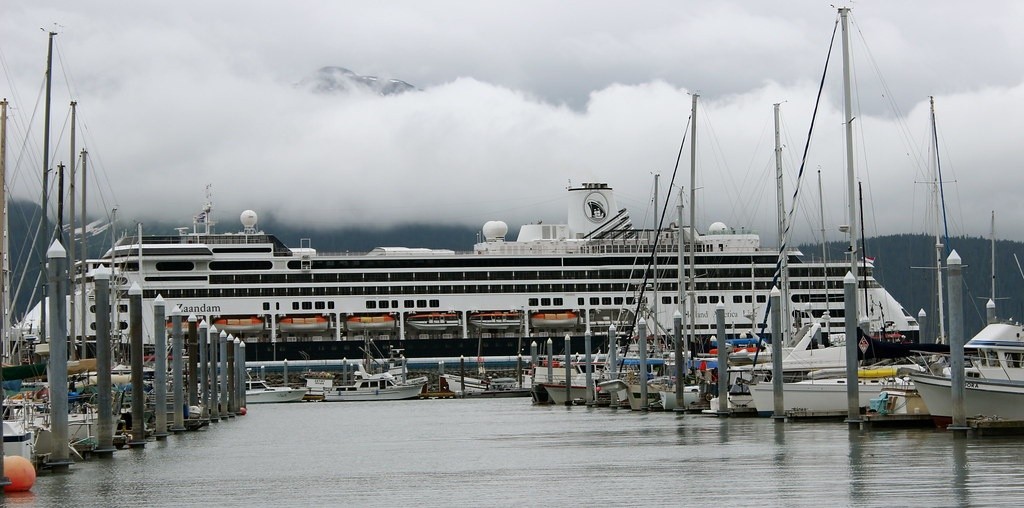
531 0 1024 435
0 21 228 462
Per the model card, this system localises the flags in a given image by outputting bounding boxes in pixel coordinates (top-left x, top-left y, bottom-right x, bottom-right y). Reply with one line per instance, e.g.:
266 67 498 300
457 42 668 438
861 256 876 264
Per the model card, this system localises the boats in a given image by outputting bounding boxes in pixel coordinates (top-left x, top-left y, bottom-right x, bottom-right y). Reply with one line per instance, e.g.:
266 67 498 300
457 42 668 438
279 313 578 332
164 311 263 336
323 329 429 401
13 178 922 376
198 365 309 404
440 368 533 399
